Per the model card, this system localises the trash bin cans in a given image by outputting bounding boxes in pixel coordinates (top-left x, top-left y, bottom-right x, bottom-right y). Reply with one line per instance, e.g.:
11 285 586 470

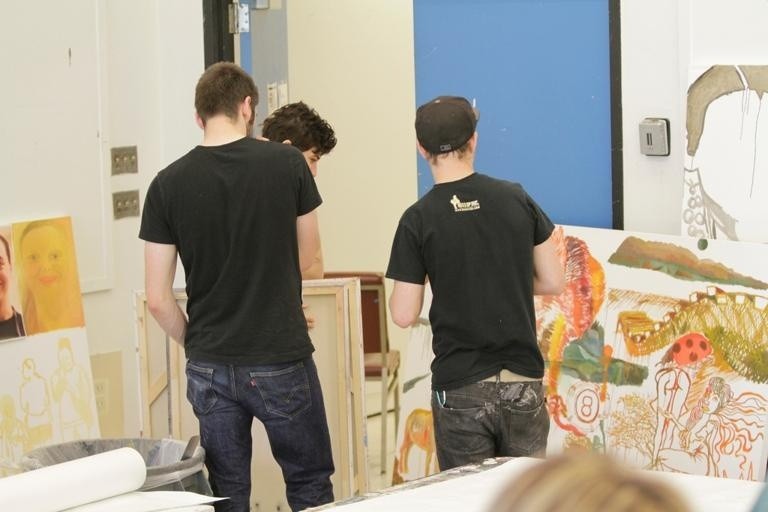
19 438 205 497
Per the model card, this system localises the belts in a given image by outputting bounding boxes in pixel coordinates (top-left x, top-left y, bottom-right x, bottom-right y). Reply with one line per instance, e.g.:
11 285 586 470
479 368 543 383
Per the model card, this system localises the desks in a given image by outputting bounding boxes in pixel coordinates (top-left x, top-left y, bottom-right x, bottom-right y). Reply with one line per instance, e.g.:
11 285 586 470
300 456 765 512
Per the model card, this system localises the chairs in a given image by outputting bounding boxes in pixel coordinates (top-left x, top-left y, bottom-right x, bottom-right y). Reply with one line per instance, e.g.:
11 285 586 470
324 272 401 473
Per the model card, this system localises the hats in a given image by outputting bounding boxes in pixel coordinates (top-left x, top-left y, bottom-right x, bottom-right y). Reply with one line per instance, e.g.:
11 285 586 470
415 94 481 154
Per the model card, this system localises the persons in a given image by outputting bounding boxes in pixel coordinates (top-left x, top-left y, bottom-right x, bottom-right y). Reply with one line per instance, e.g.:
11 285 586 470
483 447 698 512
260 98 337 331
18 225 86 334
0 235 26 340
133 60 336 512
381 94 567 472
